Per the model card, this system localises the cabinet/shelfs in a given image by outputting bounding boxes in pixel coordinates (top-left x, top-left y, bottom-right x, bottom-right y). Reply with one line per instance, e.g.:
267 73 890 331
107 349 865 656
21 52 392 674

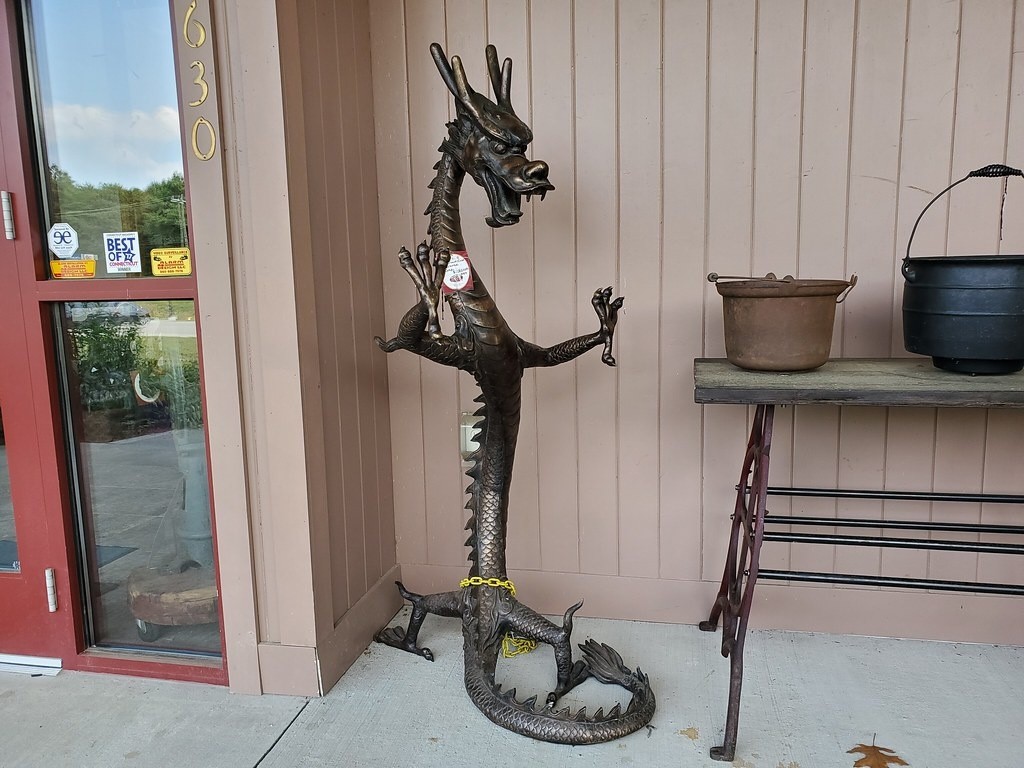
692 354 1024 768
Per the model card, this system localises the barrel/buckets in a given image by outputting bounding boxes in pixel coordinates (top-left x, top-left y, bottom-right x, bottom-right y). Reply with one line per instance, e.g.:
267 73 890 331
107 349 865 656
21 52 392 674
899 164 1024 377
703 271 860 371
